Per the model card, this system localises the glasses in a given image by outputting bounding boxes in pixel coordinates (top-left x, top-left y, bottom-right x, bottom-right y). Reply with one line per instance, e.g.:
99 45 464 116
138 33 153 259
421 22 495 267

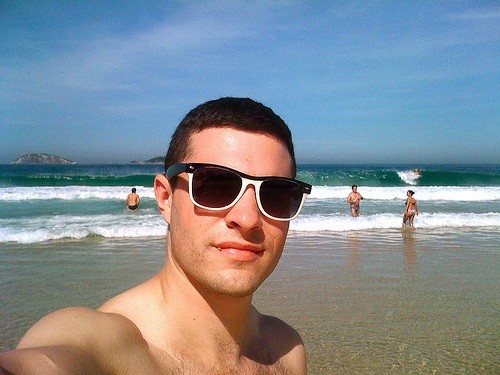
163 162 312 221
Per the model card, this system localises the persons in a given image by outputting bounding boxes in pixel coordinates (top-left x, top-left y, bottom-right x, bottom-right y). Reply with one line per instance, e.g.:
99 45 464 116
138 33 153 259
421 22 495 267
127 188 140 211
0 96 313 375
403 190 418 227
346 185 364 217
